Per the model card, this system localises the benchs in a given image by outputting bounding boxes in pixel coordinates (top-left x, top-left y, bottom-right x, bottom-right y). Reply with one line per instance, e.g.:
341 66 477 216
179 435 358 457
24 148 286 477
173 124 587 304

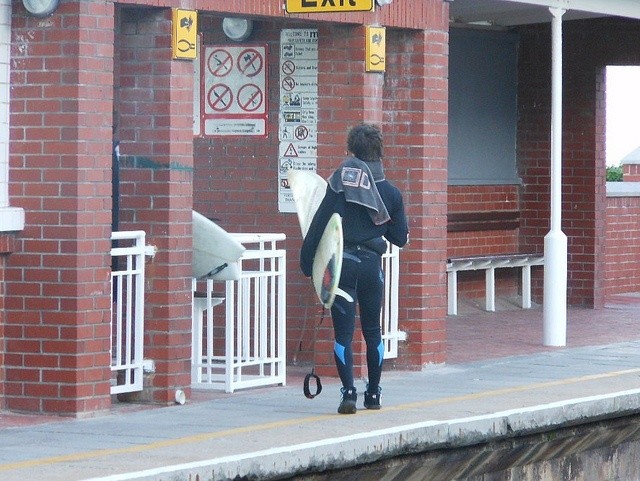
446 254 544 317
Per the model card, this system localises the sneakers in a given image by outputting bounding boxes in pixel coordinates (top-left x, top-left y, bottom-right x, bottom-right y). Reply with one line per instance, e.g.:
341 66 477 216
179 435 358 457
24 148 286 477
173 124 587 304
338 387 357 413
364 385 382 409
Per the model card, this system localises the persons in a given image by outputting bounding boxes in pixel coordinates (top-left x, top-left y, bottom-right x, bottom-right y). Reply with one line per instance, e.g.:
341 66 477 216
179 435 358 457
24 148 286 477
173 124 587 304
299 122 409 415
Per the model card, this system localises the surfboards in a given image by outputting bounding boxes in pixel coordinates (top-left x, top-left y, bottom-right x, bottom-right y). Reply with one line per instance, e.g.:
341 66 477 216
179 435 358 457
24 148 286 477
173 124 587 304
286 169 343 310
192 210 246 280
